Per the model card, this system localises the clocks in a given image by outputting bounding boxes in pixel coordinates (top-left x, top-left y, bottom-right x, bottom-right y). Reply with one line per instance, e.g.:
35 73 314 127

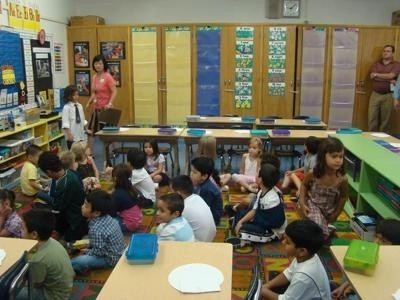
279 0 300 18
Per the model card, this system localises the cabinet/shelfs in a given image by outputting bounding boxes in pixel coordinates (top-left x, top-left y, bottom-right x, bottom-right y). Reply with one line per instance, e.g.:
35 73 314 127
262 23 331 125
326 25 397 130
1 113 66 197
194 24 263 118
130 24 194 127
66 24 134 127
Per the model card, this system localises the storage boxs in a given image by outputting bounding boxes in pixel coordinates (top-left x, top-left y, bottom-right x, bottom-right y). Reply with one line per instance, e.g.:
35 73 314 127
69 15 105 26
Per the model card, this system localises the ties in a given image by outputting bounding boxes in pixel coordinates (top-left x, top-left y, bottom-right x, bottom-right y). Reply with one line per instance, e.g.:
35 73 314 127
74 104 80 123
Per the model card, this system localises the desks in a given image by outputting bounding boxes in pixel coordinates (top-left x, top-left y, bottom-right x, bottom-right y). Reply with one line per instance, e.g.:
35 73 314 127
0 236 40 281
326 129 400 243
330 244 399 299
95 241 234 299
95 114 339 172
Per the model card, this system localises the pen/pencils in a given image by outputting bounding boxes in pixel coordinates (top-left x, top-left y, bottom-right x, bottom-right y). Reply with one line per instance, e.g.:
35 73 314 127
344 262 369 276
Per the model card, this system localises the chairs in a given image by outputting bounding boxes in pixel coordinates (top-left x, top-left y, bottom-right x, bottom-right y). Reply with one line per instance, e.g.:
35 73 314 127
242 259 264 300
113 140 306 177
1 250 32 299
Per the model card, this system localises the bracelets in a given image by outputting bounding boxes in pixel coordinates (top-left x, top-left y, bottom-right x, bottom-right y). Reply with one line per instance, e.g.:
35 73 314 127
108 102 112 106
374 73 377 78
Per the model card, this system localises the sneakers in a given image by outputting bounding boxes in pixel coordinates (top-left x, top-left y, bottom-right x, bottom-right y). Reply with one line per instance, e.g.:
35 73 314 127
222 186 229 191
241 186 246 193
225 236 252 248
251 188 258 193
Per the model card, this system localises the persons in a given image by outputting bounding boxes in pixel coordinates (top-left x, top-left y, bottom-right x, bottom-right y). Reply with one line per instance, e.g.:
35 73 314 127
0 135 400 300
60 85 95 150
365 44 399 132
391 67 399 111
83 54 117 133
